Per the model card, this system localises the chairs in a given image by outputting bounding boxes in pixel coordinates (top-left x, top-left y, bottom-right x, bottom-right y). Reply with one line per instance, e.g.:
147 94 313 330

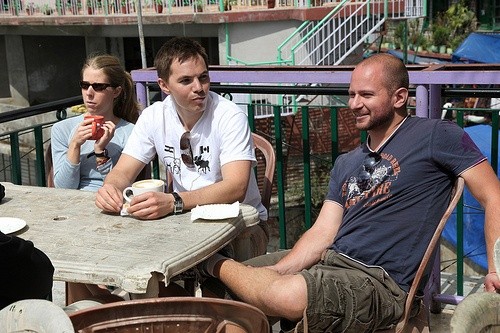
251 132 275 223
377 176 464 333
68 297 270 333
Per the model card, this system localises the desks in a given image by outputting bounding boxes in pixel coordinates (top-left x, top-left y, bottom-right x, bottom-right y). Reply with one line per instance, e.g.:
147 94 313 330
0 182 260 300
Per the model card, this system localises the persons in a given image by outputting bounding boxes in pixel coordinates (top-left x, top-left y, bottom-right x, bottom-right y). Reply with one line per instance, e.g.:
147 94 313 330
47 55 139 304
96 38 270 299
176 53 500 333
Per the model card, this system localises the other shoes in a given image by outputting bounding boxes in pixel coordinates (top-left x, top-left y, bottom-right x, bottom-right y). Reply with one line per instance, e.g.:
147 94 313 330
170 243 235 280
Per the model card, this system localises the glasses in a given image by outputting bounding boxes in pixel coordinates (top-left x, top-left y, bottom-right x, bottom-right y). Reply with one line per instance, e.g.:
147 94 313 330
356 152 381 190
180 131 196 169
79 80 117 92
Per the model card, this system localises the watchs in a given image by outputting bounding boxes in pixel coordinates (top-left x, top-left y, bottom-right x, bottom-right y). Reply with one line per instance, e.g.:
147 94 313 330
172 192 183 215
87 148 109 159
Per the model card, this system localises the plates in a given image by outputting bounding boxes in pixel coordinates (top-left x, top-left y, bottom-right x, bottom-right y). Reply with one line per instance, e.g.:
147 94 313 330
0 216 27 234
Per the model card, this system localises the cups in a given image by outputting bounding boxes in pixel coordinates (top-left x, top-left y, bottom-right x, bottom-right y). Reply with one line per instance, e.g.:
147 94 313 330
123 179 165 202
84 115 105 140
